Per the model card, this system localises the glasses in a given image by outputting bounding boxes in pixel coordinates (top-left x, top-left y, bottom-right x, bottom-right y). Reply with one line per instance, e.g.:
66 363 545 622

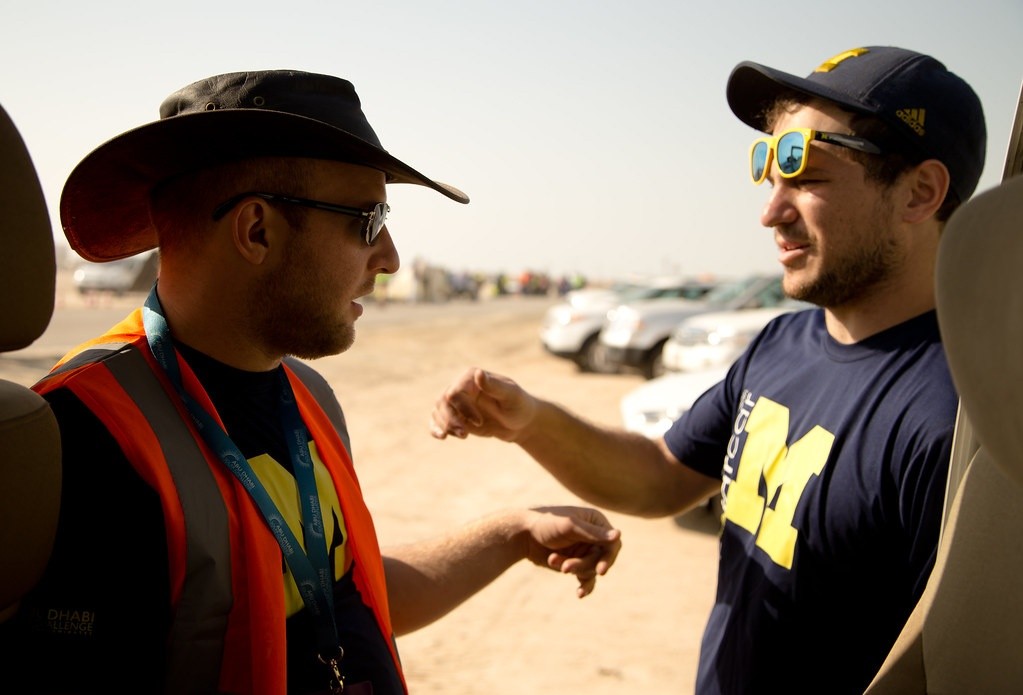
749 128 884 184
211 190 390 248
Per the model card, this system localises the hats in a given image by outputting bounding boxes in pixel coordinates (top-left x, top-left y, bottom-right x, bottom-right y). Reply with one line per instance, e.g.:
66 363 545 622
60 70 469 263
727 46 987 202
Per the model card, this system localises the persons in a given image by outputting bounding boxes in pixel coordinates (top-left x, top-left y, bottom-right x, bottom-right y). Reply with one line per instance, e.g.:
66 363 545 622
432 46 987 695
29 70 623 695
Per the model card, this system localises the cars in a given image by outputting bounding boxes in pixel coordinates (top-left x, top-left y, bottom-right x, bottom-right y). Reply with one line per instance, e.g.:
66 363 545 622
540 274 821 525
